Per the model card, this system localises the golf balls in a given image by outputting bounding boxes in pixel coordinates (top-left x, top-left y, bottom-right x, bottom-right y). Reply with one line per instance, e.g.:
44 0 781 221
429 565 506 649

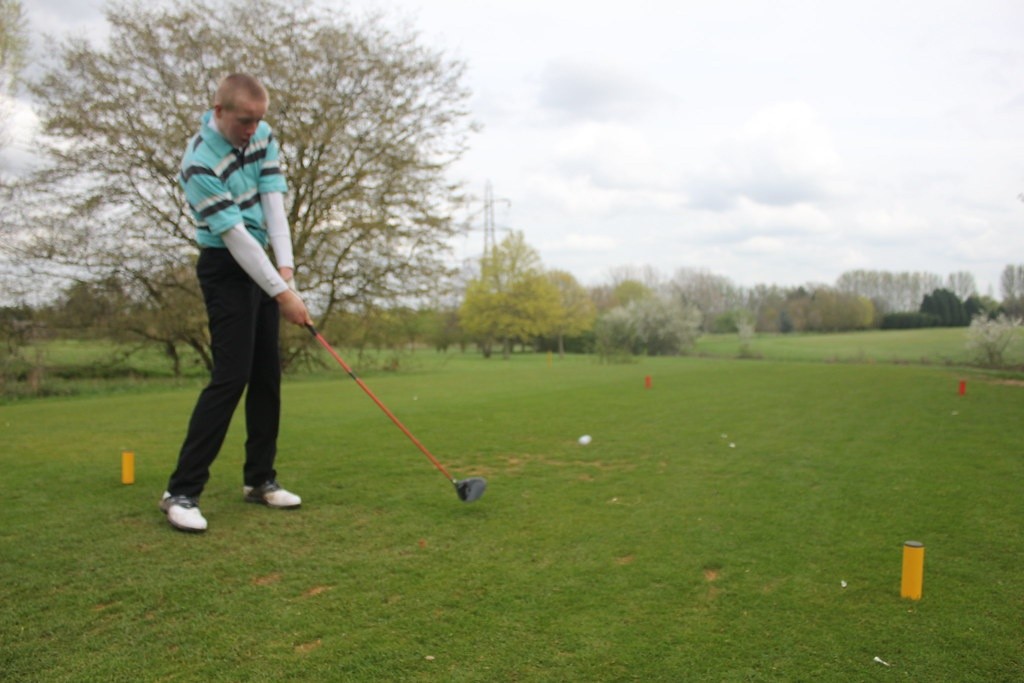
579 436 589 445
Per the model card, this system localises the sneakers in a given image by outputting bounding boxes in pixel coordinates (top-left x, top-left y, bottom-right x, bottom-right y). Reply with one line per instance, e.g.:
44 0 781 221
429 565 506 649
243 481 301 510
159 491 208 533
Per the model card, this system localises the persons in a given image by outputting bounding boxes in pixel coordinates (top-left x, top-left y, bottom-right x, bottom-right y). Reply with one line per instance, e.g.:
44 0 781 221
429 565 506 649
157 73 314 531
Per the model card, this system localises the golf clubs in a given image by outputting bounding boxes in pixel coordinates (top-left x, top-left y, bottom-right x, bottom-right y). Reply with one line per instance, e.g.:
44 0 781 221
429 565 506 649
301 321 489 504
